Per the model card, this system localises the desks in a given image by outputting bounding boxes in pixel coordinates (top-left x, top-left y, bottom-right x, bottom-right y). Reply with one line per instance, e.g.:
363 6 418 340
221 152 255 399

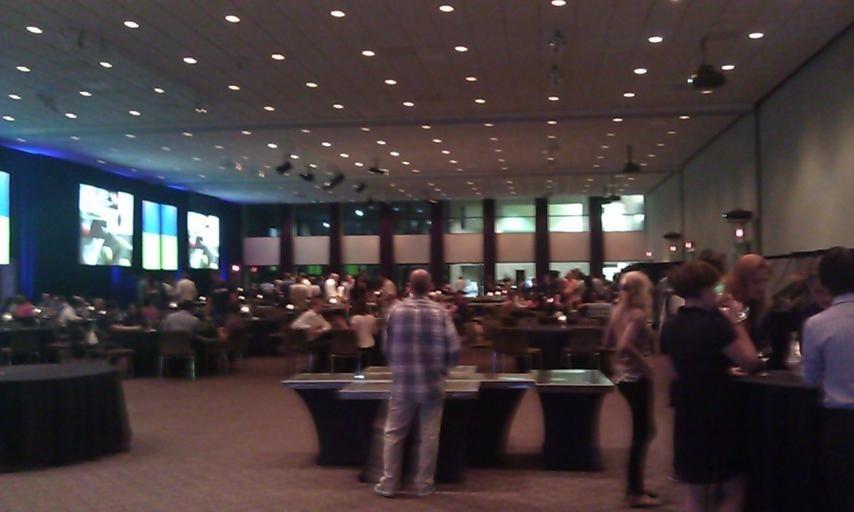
1 359 131 473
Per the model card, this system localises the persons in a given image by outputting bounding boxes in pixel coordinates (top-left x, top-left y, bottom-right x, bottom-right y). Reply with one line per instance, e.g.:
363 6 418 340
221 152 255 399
1 268 612 374
606 271 662 507
799 249 852 509
369 268 461 502
659 260 759 511
725 248 832 362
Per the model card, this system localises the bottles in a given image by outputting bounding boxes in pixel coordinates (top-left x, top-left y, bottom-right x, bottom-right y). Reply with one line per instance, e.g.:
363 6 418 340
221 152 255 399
785 330 803 378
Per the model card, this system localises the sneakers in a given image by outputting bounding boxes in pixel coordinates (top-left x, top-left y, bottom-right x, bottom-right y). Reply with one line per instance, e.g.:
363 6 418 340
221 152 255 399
417 486 434 497
374 483 394 497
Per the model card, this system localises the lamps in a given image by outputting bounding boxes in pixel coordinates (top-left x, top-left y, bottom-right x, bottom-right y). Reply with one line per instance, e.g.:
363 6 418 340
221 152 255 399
623 145 642 174
664 230 682 253
275 155 385 193
721 205 752 240
685 38 725 92
